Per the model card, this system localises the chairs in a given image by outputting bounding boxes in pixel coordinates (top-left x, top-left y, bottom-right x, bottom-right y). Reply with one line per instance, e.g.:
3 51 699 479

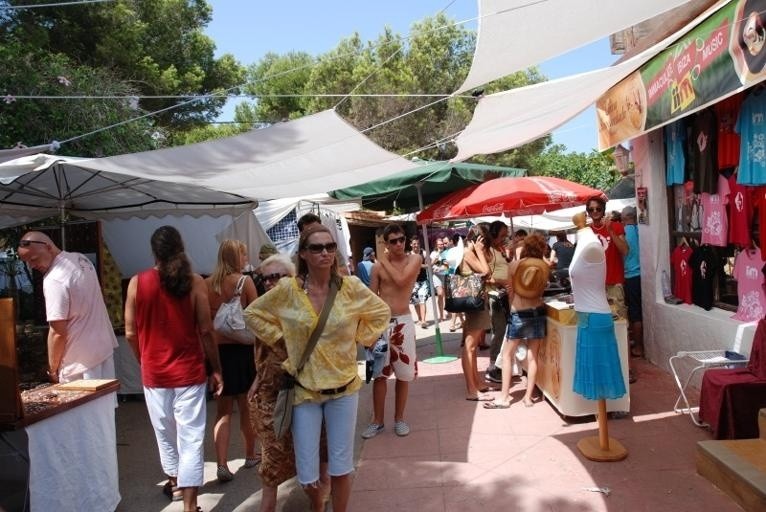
699 318 766 439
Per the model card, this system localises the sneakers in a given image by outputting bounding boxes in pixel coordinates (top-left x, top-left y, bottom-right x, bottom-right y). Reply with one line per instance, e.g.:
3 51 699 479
484 368 503 382
360 422 385 439
394 419 410 436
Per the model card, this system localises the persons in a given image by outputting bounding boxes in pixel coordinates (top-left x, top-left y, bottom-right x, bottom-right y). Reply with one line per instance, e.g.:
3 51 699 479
585 195 639 383
361 222 424 438
17 229 120 412
241 223 392 512
429 237 446 322
449 312 465 332
245 252 332 511
622 205 643 357
506 228 527 259
355 246 376 290
438 235 456 319
124 226 226 512
549 229 575 279
444 221 502 401
481 228 551 408
404 234 431 328
290 213 350 277
570 212 628 400
0 257 32 511
483 220 511 386
204 238 260 482
258 243 277 261
637 189 648 219
533 231 551 264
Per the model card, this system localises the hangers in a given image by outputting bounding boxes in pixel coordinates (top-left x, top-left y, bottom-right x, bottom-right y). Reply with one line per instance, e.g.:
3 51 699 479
677 231 690 248
699 242 708 247
752 82 765 93
733 166 739 175
746 240 758 253
717 171 720 176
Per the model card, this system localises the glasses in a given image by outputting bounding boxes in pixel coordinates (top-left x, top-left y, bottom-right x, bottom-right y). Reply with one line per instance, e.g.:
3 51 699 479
412 243 420 245
443 242 449 244
589 207 601 213
305 242 338 253
261 271 283 281
17 239 46 246
390 234 406 244
436 244 444 245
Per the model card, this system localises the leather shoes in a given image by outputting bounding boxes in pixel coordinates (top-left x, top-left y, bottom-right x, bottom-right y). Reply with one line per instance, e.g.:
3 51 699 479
455 320 465 329
244 453 262 468
449 324 456 332
421 321 427 330
217 464 237 480
417 320 421 325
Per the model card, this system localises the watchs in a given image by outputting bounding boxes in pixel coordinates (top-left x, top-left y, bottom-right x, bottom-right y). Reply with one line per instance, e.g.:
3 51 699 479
42 368 59 379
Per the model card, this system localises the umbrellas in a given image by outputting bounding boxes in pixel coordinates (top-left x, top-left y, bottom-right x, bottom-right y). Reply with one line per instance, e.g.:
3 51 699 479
414 175 610 228
326 157 531 212
0 156 260 226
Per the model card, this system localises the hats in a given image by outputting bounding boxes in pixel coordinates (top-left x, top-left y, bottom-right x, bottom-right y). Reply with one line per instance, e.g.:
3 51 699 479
510 256 551 301
622 205 636 219
363 248 373 261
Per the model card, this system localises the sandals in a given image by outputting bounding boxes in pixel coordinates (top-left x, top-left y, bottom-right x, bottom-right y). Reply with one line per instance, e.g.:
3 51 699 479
164 480 183 500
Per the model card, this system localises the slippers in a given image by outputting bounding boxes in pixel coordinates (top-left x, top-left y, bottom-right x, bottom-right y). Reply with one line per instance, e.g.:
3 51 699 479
465 393 495 401
522 395 534 407
483 400 510 410
479 384 501 393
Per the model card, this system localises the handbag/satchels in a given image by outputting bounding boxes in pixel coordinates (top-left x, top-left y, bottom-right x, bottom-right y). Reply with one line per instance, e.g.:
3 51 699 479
445 248 487 313
213 275 256 346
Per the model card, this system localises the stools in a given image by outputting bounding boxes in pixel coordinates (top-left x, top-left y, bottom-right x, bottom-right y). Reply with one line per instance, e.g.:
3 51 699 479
668 348 750 426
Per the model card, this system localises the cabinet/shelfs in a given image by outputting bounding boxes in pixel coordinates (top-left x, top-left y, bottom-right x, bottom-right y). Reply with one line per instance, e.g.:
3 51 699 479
518 291 630 422
1 297 121 512
